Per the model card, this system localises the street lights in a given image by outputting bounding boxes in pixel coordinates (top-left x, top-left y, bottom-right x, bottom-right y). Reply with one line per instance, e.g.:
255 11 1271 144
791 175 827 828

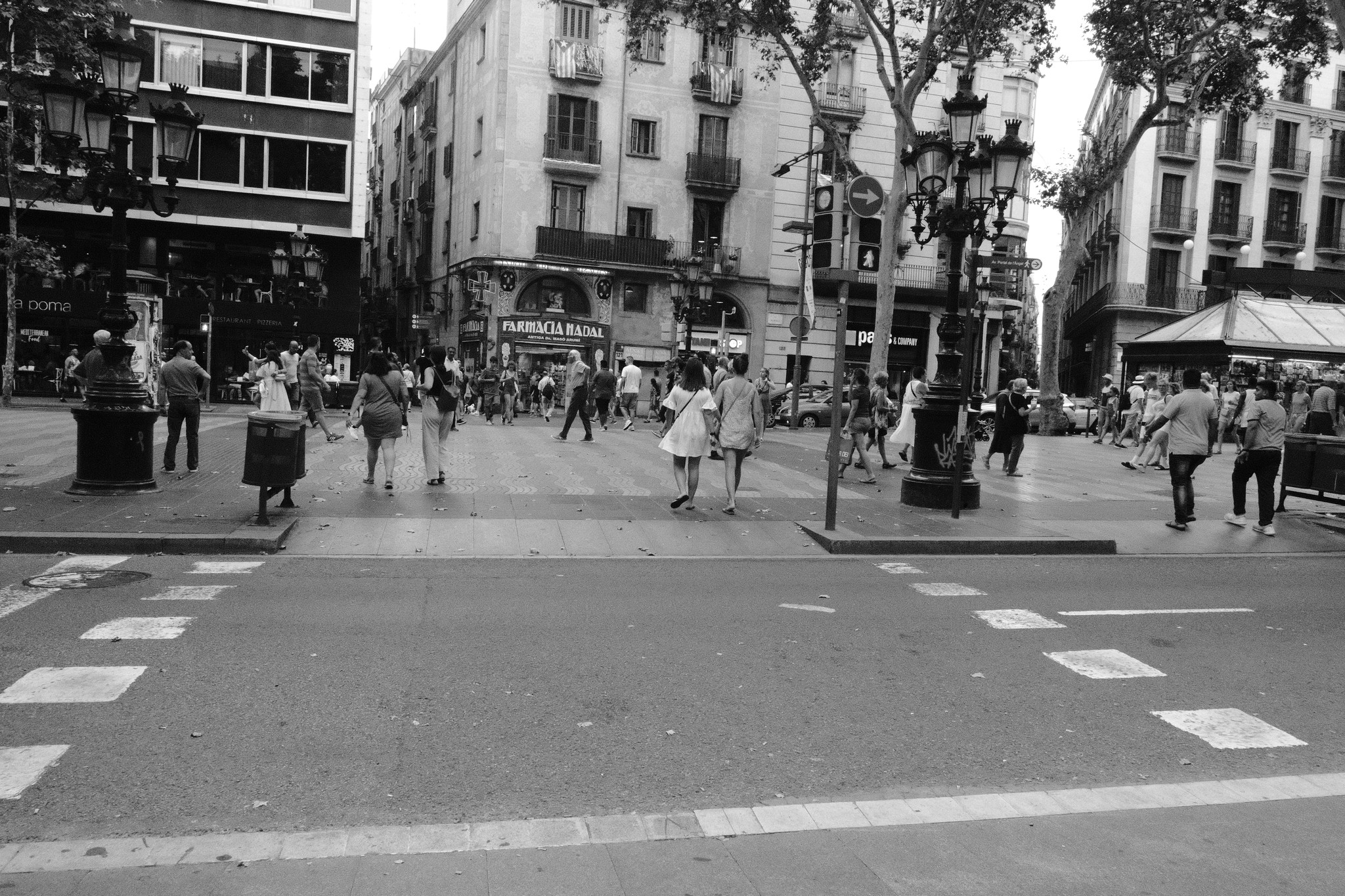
668 257 717 363
899 71 1036 510
36 9 207 496
268 222 330 357
770 124 835 430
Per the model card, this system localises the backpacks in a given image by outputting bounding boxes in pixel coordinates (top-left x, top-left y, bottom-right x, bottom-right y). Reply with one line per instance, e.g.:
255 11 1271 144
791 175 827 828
430 367 460 411
1119 387 1138 410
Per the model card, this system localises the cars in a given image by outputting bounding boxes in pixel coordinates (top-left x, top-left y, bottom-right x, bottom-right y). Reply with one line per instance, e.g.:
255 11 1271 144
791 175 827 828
977 386 1098 436
751 384 897 428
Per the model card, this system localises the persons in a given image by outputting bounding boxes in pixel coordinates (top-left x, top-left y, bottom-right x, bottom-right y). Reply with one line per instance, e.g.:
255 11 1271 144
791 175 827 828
982 378 1037 476
156 339 211 473
298 334 346 443
618 356 642 431
18 270 927 515
415 345 456 484
1070 369 1345 535
551 348 595 442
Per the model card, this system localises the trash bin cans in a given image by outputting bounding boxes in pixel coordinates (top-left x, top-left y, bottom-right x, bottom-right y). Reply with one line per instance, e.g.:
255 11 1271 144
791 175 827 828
1282 431 1345 496
241 409 307 486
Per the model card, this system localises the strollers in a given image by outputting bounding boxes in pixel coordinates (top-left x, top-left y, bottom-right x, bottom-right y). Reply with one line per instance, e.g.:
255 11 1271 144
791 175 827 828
974 421 990 442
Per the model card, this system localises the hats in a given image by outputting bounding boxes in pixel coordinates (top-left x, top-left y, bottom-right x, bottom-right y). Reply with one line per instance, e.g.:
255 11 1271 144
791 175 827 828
1102 373 1113 381
1132 375 1145 383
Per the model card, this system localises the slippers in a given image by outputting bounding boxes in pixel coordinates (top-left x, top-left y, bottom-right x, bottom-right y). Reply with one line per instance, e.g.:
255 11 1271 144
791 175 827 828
980 456 990 470
722 507 735 514
670 495 694 510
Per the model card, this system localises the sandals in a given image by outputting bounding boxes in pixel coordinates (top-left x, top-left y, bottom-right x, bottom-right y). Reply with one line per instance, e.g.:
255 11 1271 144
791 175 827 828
427 472 445 485
838 449 913 483
1165 515 1196 531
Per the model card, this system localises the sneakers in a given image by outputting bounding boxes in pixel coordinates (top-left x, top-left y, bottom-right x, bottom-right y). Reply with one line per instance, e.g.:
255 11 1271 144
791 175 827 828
1224 513 1276 536
326 432 345 442
589 415 660 431
652 431 662 438
1092 438 1242 472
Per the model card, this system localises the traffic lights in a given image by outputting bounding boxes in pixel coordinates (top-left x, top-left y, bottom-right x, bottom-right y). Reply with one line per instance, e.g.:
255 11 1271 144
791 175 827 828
410 314 417 330
843 212 883 273
811 182 843 269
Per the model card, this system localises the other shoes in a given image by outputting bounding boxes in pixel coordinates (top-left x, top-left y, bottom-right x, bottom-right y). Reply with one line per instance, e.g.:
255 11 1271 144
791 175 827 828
1005 467 1024 477
189 465 198 472
401 425 407 430
312 420 319 428
81 399 87 403
577 437 595 443
550 434 567 442
408 409 411 412
450 414 550 432
1002 467 1018 472
709 451 724 461
160 466 174 473
363 475 374 484
384 478 394 489
743 451 752 458
60 398 67 403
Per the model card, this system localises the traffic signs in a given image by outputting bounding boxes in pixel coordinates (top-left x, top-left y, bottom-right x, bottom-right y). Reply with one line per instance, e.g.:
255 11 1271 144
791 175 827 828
962 248 973 278
978 255 1043 270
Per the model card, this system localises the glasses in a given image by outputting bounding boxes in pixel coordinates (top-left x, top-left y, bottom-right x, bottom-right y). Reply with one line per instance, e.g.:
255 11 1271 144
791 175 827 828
291 347 299 350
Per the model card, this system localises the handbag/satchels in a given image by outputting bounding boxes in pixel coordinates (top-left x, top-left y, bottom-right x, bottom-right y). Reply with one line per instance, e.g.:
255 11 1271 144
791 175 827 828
541 377 555 400
498 385 504 391
514 398 524 413
500 393 505 402
248 357 264 383
1021 417 1032 435
1220 408 1229 417
400 409 408 426
824 389 897 466
274 373 286 381
